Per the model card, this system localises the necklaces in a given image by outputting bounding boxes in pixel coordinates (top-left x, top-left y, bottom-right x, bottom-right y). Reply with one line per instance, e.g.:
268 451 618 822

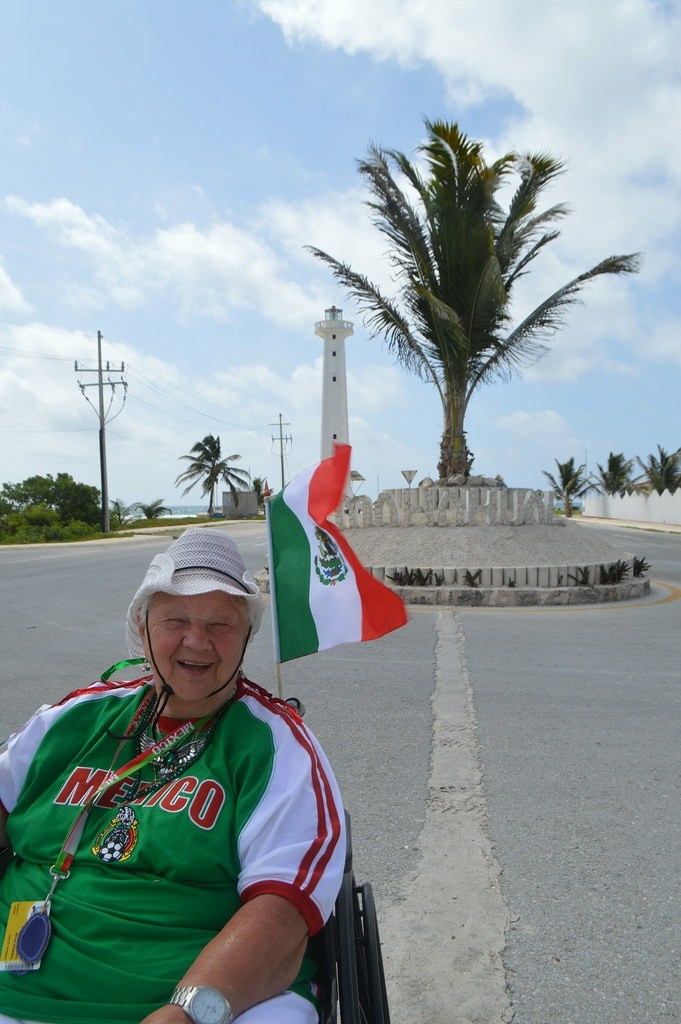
126 694 228 800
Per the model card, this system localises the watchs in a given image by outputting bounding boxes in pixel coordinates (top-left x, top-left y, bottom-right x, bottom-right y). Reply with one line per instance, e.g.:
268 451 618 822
169 984 235 1024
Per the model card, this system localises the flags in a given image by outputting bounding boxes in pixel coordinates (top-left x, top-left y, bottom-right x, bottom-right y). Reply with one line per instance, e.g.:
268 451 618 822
267 444 411 663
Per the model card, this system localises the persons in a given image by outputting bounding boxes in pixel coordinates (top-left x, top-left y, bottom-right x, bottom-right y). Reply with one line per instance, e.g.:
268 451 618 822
0 528 346 1024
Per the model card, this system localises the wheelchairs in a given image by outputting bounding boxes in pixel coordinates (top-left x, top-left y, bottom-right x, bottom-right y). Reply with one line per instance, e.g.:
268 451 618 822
286 696 390 1024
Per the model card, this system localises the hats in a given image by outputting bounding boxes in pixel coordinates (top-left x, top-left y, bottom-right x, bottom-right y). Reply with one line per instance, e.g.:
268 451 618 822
105 527 265 668
